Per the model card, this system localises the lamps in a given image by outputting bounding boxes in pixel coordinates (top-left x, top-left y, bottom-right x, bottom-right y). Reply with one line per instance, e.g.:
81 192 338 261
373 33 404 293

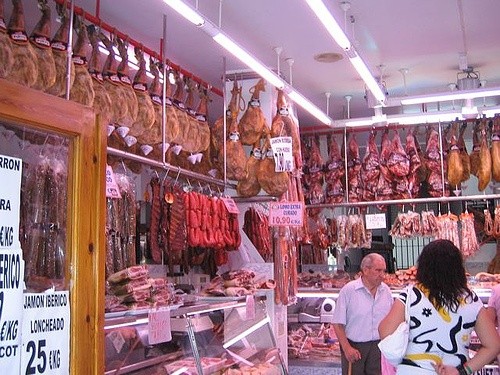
162 0 500 130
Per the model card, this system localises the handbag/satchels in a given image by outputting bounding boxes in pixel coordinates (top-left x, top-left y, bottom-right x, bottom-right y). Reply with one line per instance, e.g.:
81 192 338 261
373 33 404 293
377 285 413 367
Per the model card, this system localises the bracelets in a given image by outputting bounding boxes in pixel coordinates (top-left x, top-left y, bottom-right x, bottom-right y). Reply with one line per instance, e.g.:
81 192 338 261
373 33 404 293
456 363 472 375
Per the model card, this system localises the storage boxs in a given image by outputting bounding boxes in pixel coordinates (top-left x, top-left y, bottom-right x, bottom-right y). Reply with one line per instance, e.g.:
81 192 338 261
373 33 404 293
164 356 228 375
192 274 210 287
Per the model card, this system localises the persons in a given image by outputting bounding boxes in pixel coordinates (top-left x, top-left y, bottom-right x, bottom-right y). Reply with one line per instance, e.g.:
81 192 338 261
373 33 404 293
378 240 500 374
332 253 395 375
486 284 500 327
